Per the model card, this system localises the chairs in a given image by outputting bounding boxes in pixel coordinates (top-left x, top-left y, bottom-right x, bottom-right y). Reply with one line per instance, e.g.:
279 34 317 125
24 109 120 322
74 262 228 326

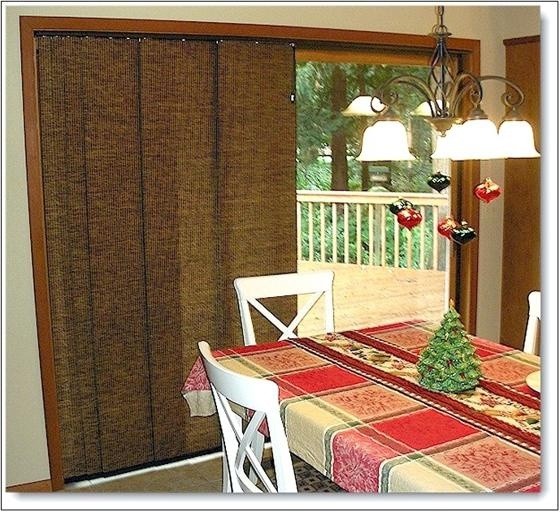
198 341 298 495
521 290 542 354
232 269 336 345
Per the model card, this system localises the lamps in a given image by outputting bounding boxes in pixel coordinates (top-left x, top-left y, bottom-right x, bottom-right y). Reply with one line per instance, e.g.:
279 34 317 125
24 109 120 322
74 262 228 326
338 5 541 164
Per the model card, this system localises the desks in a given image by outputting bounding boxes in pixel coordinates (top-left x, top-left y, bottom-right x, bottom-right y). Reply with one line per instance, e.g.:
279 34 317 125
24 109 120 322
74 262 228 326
185 318 540 494
